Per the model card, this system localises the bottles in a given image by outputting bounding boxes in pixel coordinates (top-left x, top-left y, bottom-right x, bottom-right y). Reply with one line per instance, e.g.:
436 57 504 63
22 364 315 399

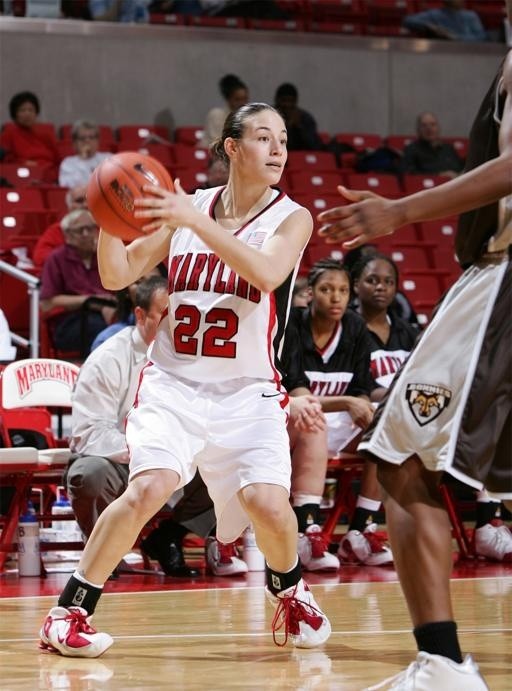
16 513 43 579
27 499 37 517
51 496 73 532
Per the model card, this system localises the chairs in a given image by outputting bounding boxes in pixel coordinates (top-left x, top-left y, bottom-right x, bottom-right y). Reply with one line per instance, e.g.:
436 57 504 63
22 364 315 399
0 0 512 574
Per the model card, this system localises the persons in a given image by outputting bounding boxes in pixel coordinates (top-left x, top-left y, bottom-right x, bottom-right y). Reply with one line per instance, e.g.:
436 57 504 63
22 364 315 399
344 243 421 329
405 113 461 174
39 209 118 351
1 89 67 169
0 0 290 27
203 262 393 574
32 186 87 270
315 44 510 691
59 116 116 187
269 83 323 152
201 73 249 146
89 262 167 353
400 1 512 45
38 100 330 659
64 278 217 582
353 256 512 564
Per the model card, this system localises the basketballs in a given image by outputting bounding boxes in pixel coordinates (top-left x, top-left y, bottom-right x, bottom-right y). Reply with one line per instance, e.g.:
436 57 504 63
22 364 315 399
89 153 169 237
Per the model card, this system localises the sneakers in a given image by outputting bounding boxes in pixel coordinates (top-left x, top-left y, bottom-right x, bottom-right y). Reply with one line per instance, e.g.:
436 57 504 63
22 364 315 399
38 606 113 659
471 519 512 563
263 576 331 649
297 524 393 571
207 537 249 576
364 652 488 690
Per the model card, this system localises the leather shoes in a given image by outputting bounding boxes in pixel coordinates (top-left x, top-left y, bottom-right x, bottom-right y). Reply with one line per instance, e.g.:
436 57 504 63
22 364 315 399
139 528 201 578
